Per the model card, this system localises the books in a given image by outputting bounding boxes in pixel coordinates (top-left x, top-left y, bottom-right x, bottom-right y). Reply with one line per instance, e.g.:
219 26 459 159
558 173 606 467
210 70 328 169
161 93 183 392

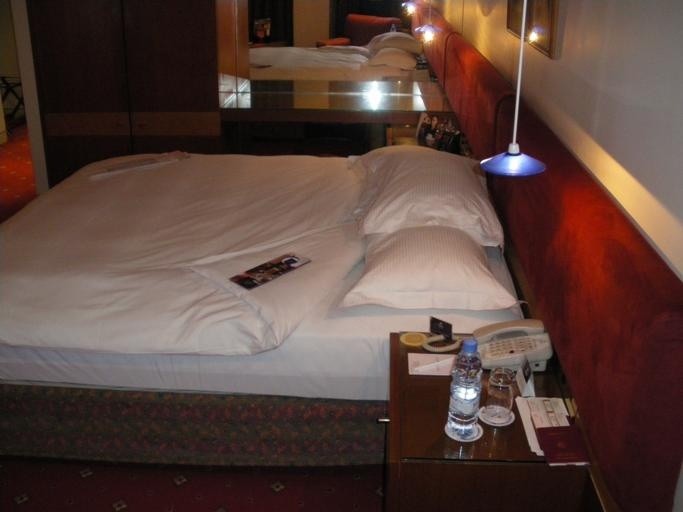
228 252 312 291
534 426 590 466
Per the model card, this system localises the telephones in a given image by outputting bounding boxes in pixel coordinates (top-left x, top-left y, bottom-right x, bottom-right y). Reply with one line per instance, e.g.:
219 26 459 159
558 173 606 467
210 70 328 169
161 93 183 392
472 319 554 373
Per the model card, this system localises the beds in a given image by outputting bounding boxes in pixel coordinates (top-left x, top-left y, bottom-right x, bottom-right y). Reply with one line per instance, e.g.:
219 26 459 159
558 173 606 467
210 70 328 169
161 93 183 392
0 154 524 470
247 46 437 81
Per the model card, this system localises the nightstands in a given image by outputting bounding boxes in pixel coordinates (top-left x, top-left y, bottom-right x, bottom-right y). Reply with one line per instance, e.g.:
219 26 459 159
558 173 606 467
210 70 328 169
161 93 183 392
387 332 589 510
386 125 473 157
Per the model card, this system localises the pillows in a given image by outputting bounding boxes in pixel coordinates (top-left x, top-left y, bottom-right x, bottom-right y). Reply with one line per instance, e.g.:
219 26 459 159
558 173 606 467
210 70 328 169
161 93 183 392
339 226 518 316
369 47 418 71
367 32 422 54
356 145 506 247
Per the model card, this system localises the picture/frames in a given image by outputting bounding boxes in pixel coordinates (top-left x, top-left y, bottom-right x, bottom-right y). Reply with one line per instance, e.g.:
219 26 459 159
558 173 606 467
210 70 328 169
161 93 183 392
504 0 557 62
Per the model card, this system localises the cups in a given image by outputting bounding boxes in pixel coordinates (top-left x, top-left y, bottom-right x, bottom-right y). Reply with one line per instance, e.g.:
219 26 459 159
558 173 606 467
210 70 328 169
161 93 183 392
484 367 514 420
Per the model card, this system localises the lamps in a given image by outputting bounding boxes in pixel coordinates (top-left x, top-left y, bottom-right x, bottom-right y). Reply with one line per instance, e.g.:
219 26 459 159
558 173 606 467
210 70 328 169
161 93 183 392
525 1 544 45
403 2 416 17
482 1 546 176
417 0 443 44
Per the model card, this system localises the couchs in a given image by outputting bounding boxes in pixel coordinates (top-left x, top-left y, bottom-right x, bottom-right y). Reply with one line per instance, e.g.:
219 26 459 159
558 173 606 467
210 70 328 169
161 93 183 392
315 14 404 48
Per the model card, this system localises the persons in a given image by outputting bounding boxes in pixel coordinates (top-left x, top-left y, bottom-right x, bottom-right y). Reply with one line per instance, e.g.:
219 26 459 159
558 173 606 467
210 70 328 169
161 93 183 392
424 116 437 136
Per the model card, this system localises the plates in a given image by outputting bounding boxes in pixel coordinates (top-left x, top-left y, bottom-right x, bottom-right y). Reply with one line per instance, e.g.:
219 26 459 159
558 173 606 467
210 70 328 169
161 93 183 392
445 423 483 443
479 405 515 426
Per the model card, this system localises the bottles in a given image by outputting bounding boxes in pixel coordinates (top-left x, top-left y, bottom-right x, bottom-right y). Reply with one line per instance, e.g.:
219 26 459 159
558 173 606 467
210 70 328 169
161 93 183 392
390 24 396 31
448 337 483 440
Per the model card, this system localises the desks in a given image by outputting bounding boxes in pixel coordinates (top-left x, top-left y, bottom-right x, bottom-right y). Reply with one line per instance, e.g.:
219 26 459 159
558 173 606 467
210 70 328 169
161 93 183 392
223 85 456 149
